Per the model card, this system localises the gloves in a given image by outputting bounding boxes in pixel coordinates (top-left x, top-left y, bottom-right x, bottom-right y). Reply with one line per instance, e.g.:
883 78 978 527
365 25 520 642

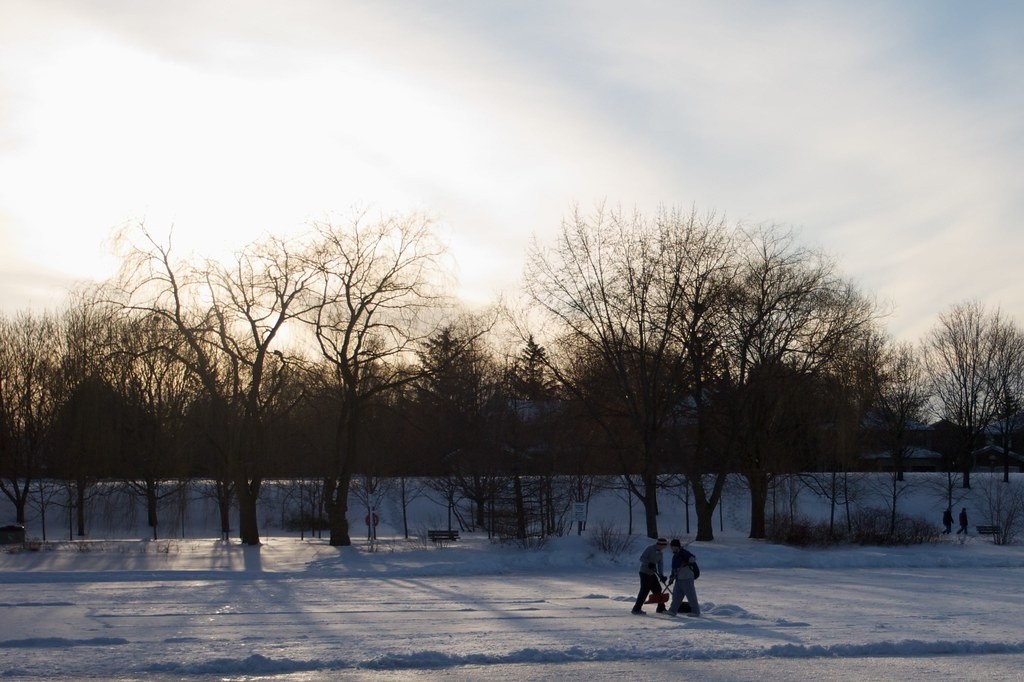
649 563 656 570
669 580 673 585
660 575 666 583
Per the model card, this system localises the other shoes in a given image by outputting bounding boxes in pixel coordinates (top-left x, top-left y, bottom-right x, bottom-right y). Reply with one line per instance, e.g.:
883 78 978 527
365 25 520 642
656 607 667 613
631 610 646 615
663 610 677 616
687 613 700 617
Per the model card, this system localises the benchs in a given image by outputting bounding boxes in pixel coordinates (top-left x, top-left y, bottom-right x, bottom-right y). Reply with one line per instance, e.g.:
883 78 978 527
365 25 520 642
428 530 460 543
976 526 1003 536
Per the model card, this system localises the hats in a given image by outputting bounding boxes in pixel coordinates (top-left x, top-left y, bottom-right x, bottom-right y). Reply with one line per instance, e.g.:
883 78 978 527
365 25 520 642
657 538 667 545
671 539 680 546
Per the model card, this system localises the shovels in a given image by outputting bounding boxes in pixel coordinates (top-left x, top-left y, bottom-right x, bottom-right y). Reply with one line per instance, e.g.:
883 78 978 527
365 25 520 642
643 562 683 605
654 572 691 613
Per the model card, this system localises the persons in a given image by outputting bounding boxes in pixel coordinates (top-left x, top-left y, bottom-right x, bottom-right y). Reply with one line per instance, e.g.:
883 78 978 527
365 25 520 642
942 508 955 534
631 538 668 615
956 508 969 534
663 538 702 617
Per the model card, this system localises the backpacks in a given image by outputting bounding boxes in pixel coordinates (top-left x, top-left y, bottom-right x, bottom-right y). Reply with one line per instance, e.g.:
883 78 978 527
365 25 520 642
681 550 699 580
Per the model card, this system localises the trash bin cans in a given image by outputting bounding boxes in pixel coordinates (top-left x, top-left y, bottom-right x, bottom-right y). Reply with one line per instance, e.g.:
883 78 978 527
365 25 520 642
0 525 25 544
